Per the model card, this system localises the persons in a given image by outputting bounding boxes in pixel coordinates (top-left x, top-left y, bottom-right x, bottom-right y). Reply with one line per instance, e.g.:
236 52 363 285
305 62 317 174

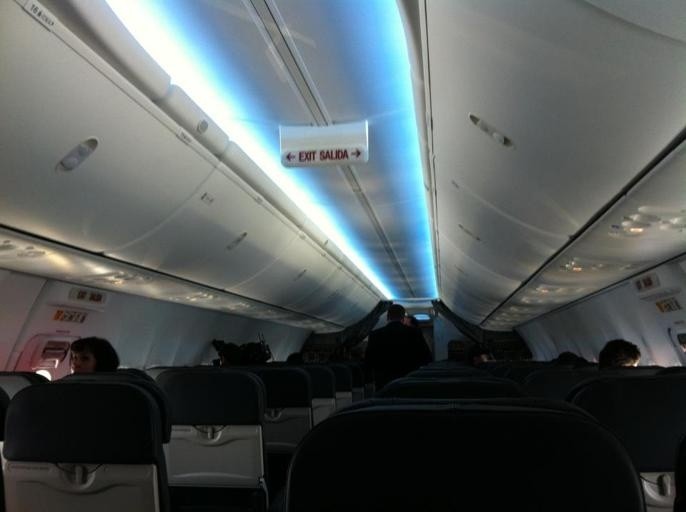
71 337 119 375
370 304 422 372
468 335 504 372
404 315 431 365
599 340 640 369
218 342 244 368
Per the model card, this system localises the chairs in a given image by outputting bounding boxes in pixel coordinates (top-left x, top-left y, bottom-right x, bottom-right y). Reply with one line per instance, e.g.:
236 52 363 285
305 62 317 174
1 351 686 511
